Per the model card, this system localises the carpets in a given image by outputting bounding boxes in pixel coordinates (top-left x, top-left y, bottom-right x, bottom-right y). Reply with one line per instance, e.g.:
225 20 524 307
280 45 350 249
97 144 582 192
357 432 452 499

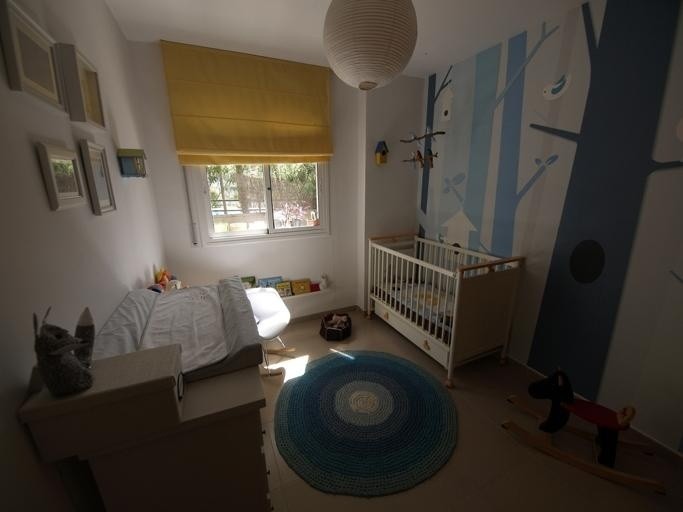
272 348 462 500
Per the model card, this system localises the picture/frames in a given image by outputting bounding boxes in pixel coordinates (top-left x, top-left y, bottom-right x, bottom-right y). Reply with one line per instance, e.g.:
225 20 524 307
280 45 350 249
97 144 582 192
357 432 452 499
54 40 110 137
75 136 119 219
2 1 71 119
30 137 91 216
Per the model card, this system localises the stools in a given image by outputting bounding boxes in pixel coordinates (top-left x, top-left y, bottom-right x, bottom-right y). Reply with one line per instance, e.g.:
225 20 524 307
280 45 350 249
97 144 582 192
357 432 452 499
243 285 300 378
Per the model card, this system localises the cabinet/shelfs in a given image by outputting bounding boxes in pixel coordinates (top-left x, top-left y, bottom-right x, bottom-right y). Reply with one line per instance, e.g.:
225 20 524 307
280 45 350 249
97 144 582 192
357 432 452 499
79 346 274 511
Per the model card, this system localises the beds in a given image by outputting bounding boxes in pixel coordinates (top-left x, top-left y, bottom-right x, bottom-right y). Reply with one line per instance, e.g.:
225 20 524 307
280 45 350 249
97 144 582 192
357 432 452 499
362 230 530 391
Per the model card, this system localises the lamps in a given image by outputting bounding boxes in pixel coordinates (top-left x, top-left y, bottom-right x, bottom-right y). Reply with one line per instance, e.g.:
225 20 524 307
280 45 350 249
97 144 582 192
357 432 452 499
319 0 423 96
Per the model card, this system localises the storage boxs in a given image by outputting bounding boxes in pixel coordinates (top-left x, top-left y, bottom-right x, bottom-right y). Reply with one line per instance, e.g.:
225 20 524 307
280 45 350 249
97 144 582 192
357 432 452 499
14 344 194 470
